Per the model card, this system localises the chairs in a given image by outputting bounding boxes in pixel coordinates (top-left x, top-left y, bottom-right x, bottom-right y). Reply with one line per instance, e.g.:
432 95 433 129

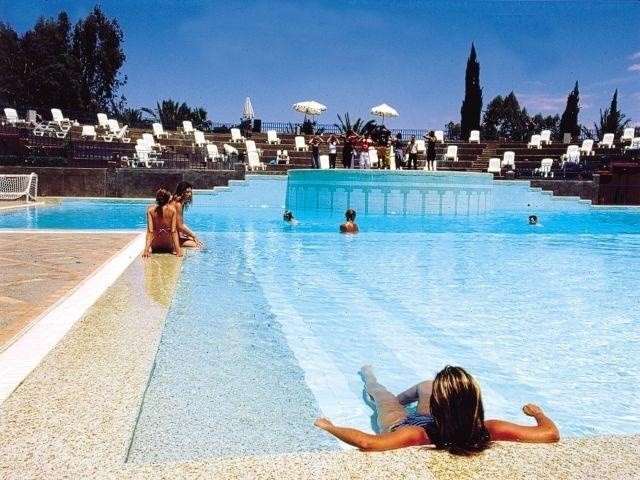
433 130 444 145
485 127 640 178
413 139 428 156
173 120 267 173
367 148 384 168
443 145 459 163
120 122 172 169
468 130 481 145
293 135 310 152
274 149 290 165
265 130 283 144
1 108 130 146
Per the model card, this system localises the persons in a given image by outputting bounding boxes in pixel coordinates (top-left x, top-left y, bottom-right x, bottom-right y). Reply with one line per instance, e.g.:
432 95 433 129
309 135 323 169
315 364 560 455
342 131 437 171
141 187 181 259
327 135 340 169
529 215 540 225
283 211 295 224
168 182 204 248
340 209 358 232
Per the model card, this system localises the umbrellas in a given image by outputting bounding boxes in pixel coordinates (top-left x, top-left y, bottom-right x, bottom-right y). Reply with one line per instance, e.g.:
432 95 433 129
243 97 254 118
292 101 327 121
371 103 399 124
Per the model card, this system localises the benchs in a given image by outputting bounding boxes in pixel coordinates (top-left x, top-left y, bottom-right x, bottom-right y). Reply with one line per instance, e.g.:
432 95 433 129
0 172 38 202
